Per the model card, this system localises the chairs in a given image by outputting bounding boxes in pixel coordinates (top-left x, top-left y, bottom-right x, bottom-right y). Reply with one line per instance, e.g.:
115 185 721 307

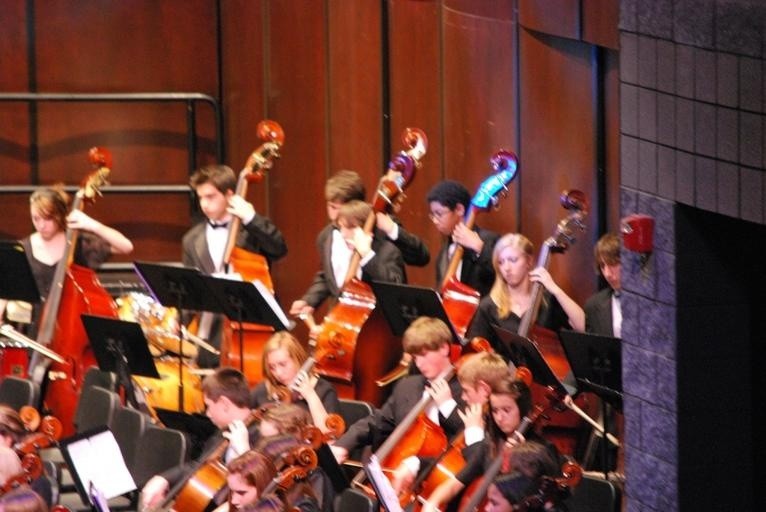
75 383 120 441
544 472 620 512
337 395 376 465
109 405 145 480
135 426 186 488
0 374 42 417
334 487 380 512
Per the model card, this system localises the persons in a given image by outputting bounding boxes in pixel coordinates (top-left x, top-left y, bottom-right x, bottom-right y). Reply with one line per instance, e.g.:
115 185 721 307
421 381 570 512
142 368 263 510
392 353 509 497
249 332 351 466
224 451 296 512
290 170 431 318
261 440 320 512
171 166 289 370
308 199 408 346
328 316 469 467
0 405 52 507
553 232 624 411
427 183 500 297
0 188 133 439
486 473 544 512
464 233 587 359
212 406 331 512
503 442 559 512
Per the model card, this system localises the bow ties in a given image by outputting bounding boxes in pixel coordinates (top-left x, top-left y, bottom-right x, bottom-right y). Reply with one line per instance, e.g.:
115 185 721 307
332 224 339 231
427 209 453 221
614 293 621 299
206 220 228 228
422 379 431 387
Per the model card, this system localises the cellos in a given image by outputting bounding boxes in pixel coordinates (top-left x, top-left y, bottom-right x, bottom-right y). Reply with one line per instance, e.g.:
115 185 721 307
351 334 496 512
0 453 43 501
263 446 319 496
274 425 323 474
182 119 283 394
380 365 532 512
454 383 566 512
313 127 428 411
149 398 274 512
435 146 519 343
28 144 129 408
516 187 584 397
269 328 342 406
555 455 583 488
318 412 345 445
9 416 63 457
19 406 40 431
